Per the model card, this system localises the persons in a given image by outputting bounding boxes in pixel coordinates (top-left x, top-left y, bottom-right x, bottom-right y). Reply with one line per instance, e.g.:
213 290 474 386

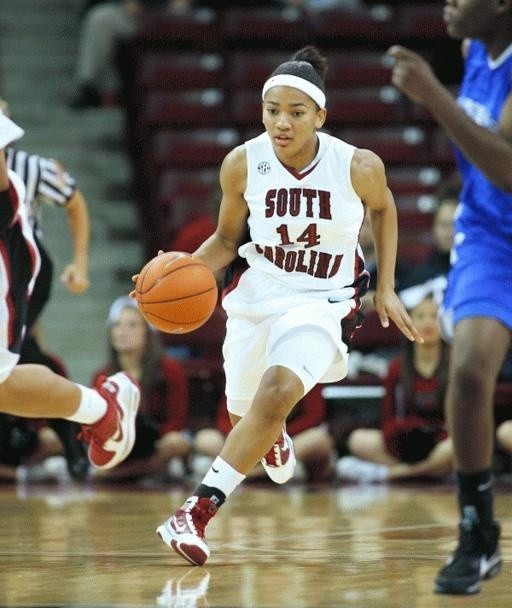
128 47 429 563
67 0 191 110
2 108 91 487
386 0 512 594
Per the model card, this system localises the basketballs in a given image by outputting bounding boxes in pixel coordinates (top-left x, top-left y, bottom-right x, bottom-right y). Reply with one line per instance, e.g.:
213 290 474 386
136 251 219 335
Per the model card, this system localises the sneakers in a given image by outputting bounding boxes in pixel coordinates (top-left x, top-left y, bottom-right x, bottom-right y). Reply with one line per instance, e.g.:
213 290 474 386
164 455 215 481
154 493 219 568
259 418 297 487
20 454 70 487
434 517 504 596
335 455 389 483
76 367 143 470
